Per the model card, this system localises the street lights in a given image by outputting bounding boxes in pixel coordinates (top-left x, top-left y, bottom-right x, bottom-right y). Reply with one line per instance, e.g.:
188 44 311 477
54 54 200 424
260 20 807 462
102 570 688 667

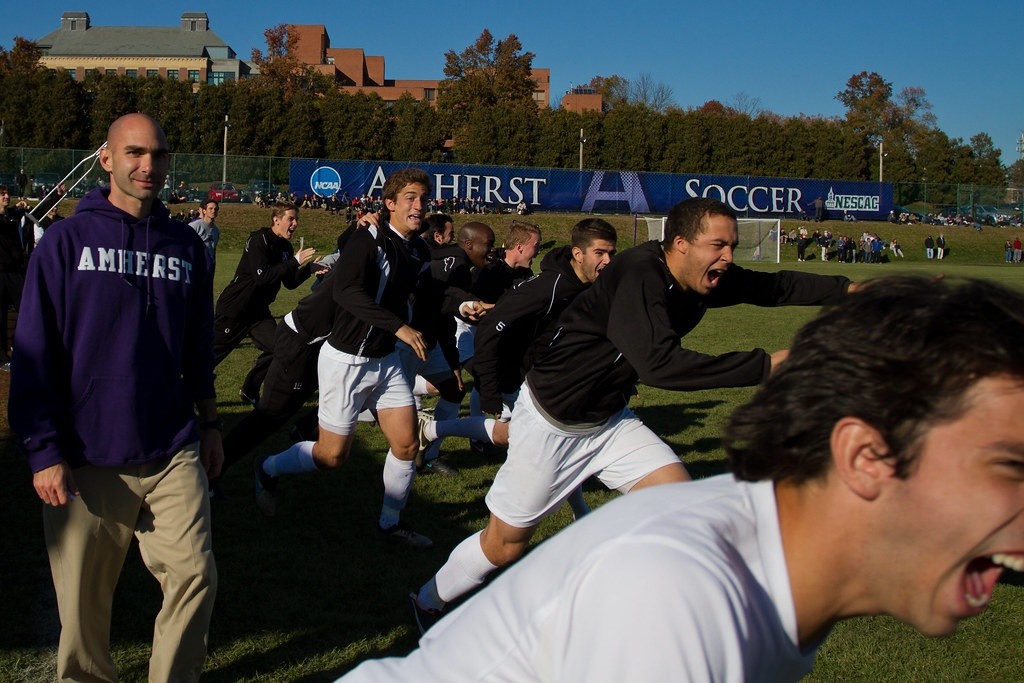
222 114 233 186
879 142 888 182
579 126 588 169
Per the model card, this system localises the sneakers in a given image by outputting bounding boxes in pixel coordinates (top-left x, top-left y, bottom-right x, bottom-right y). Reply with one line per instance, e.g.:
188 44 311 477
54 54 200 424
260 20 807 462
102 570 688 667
371 515 435 551
416 413 431 451
251 455 280 505
419 457 458 477
238 389 260 408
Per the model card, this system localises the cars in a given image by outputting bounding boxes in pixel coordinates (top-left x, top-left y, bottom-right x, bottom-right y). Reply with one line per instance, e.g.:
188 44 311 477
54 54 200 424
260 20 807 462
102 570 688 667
967 204 1012 227
937 207 987 225
208 182 240 203
236 189 252 204
249 180 280 198
1009 210 1024 226
0 172 22 196
71 179 100 198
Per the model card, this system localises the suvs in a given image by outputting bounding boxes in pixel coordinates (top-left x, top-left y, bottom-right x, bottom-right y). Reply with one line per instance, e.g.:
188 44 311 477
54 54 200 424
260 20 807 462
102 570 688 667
29 172 61 198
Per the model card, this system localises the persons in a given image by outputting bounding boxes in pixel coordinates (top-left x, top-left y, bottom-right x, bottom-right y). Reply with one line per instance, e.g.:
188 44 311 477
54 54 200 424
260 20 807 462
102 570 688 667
326 265 1024 683
1013 237 1022 263
0 113 219 683
1005 239 1012 263
924 235 934 259
936 232 945 259
211 164 884 632
843 209 857 222
769 223 905 265
799 209 812 221
807 195 824 223
887 210 1024 231
